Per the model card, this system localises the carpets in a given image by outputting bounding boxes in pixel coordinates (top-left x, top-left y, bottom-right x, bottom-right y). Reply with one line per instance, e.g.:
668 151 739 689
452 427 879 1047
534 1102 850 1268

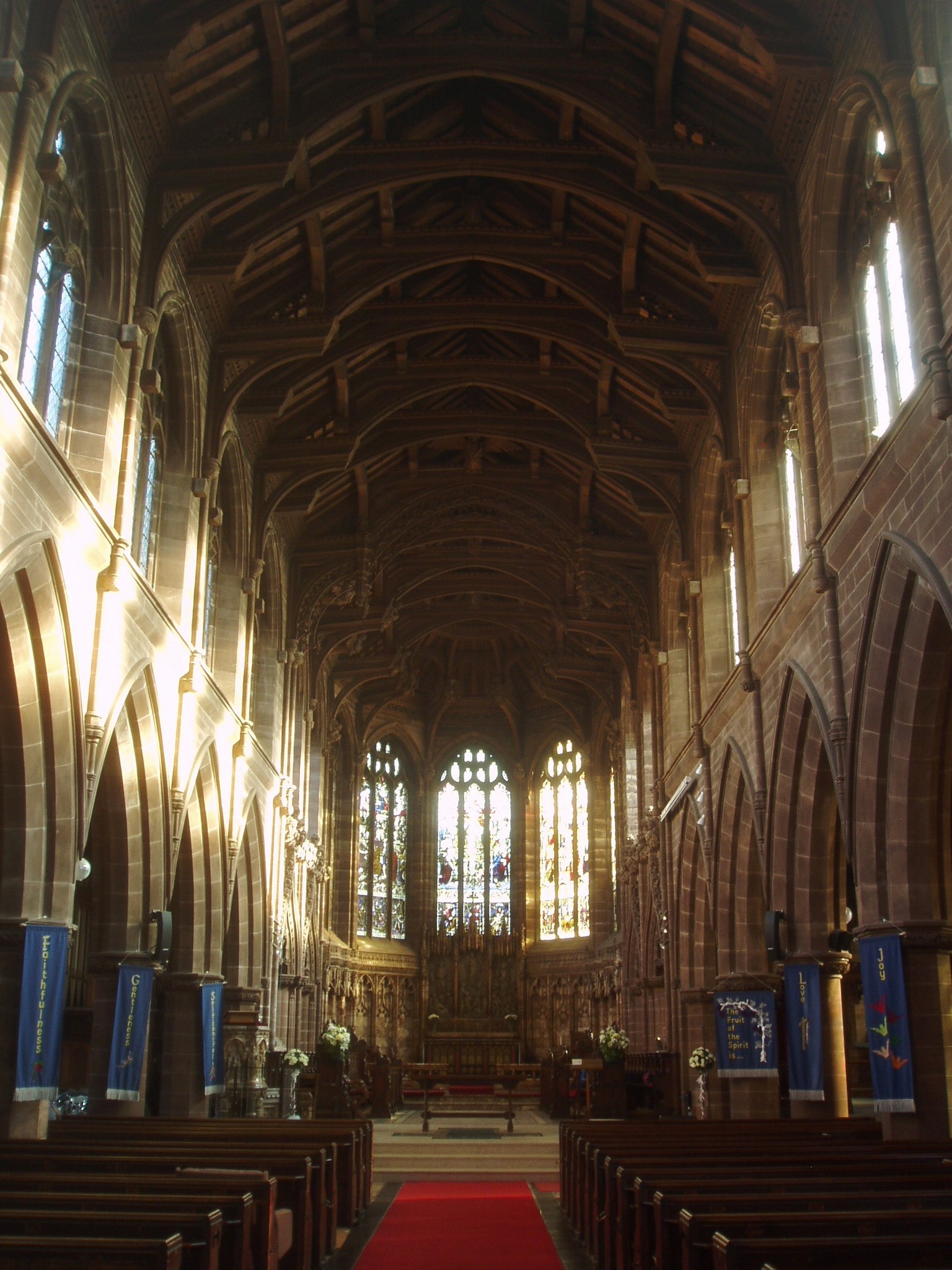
433 1127 502 1139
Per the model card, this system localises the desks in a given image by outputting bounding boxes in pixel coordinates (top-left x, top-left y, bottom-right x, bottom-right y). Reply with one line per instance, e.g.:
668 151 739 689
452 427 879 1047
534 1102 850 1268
426 1032 519 1075
495 1064 541 1079
405 1063 449 1076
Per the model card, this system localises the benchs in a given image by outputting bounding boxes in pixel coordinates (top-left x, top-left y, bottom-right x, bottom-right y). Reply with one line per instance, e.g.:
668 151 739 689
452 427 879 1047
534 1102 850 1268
265 1051 403 1119
558 1120 952 1270
1 1116 374 1270
538 1046 671 1118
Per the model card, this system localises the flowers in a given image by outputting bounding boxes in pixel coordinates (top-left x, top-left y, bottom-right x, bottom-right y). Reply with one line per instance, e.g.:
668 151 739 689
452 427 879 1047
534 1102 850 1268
689 1047 716 1070
599 1027 629 1063
505 1015 517 1026
321 1019 350 1061
428 1014 439 1025
284 1049 309 1071
